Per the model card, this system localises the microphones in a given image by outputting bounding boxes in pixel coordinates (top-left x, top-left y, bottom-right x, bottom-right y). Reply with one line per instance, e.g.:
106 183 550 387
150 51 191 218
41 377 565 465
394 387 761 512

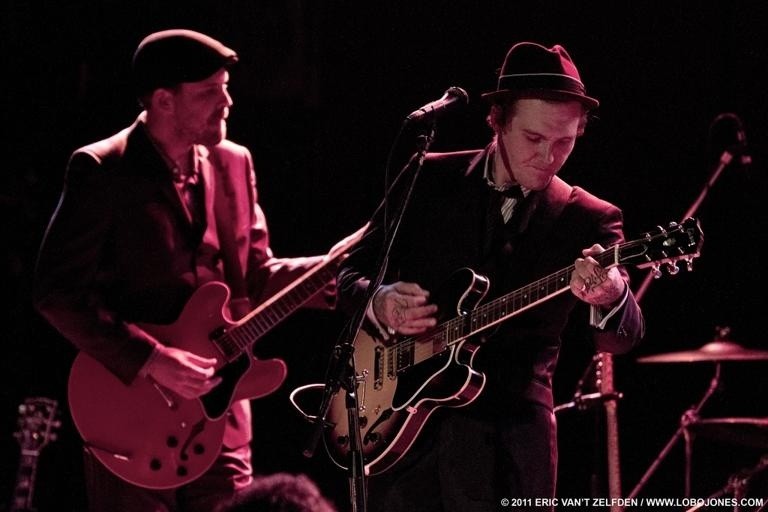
406 86 470 131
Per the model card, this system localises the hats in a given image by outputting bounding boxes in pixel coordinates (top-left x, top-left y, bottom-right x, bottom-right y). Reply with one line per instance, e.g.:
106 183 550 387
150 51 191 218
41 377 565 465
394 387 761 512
477 39 603 113
131 26 242 88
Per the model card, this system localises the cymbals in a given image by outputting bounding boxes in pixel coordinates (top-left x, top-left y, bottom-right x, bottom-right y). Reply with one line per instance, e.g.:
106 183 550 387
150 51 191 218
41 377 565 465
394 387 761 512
697 418 768 425
636 342 767 364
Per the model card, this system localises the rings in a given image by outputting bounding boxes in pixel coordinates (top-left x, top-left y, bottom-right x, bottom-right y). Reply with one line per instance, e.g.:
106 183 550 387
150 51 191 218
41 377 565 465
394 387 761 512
577 283 588 293
183 376 190 387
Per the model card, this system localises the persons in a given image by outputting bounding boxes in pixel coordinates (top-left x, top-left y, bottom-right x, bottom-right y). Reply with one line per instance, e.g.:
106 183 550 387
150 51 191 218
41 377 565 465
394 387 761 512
27 27 362 510
334 40 650 511
210 471 337 511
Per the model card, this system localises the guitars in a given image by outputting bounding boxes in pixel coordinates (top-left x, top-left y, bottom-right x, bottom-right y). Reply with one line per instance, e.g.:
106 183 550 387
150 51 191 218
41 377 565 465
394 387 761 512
67 219 385 490
322 217 704 476
11 398 60 511
